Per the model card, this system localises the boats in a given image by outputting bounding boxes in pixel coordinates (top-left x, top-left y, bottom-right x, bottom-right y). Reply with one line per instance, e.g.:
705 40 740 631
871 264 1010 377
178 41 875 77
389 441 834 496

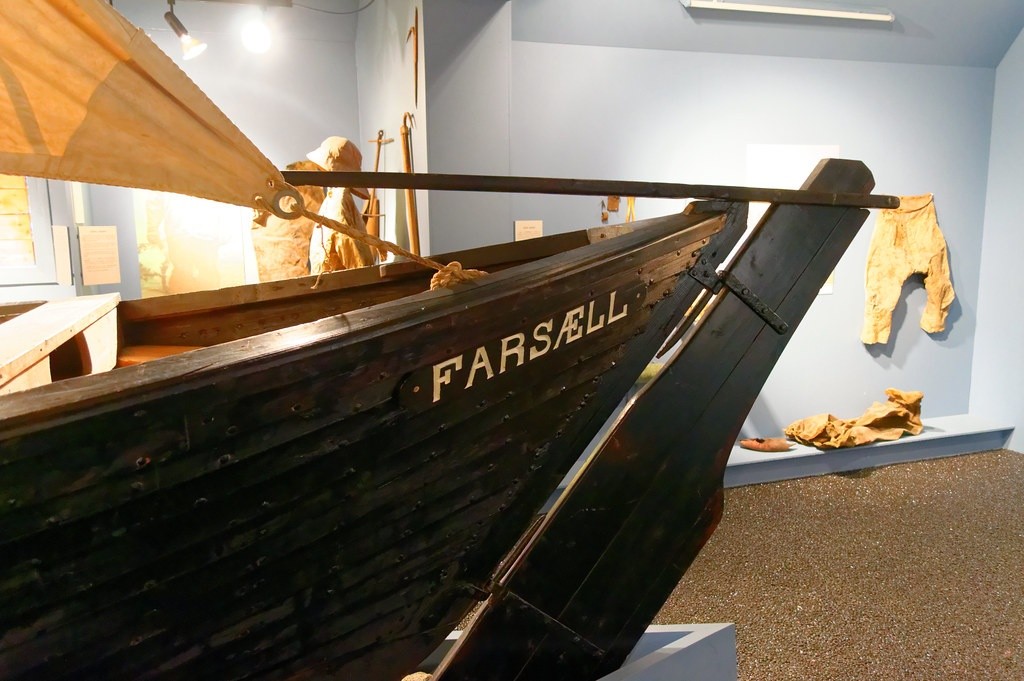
0 156 899 681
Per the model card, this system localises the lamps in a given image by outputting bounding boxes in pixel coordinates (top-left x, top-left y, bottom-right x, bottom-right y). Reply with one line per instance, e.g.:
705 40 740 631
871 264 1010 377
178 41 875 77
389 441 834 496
163 10 208 61
679 0 896 23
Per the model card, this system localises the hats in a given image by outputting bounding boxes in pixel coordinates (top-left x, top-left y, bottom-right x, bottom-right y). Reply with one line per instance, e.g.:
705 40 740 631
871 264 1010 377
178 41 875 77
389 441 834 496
307 135 370 201
